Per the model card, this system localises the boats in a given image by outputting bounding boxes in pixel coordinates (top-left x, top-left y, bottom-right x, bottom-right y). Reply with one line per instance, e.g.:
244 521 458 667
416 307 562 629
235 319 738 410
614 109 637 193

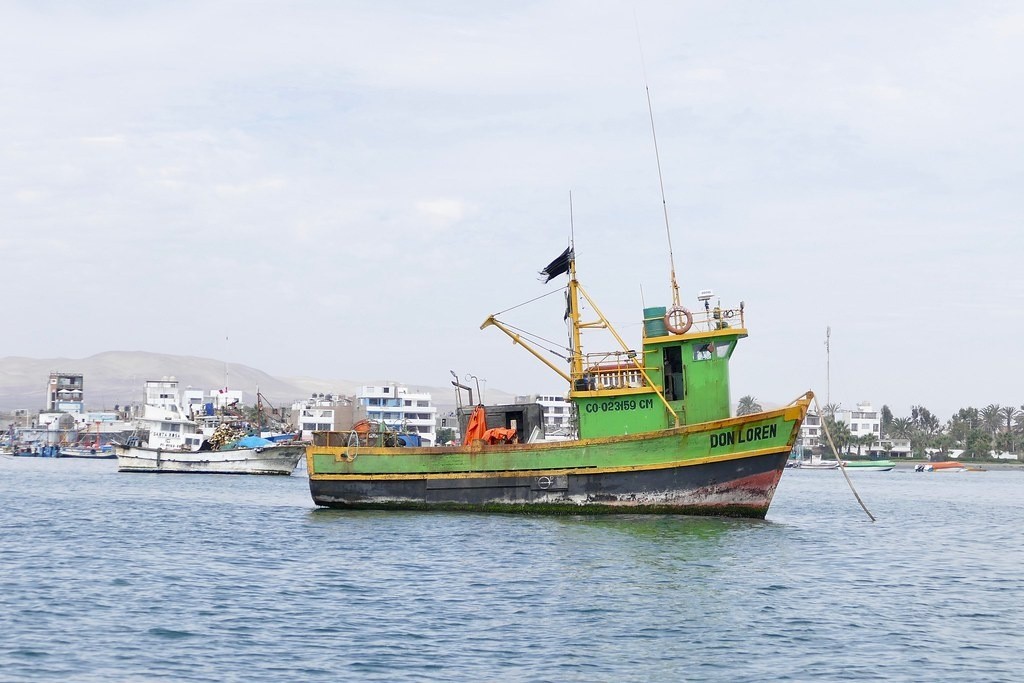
110 384 311 476
59 420 122 458
303 83 813 520
915 461 987 473
787 460 895 470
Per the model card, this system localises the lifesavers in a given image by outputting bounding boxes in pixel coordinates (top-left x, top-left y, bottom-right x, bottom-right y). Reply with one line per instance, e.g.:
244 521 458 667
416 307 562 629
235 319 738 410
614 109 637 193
663 306 693 335
